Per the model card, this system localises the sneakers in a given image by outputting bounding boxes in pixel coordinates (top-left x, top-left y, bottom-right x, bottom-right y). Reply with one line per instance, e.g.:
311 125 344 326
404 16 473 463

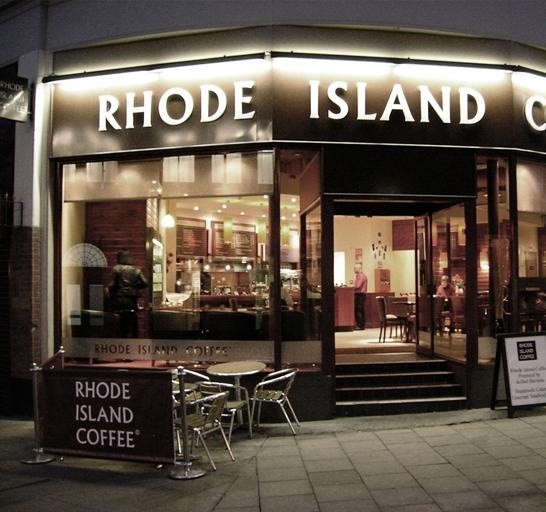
355 326 364 329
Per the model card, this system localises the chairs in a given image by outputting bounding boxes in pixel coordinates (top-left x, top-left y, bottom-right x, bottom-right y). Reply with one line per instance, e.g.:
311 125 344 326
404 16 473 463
155 310 305 340
165 366 299 471
375 287 544 340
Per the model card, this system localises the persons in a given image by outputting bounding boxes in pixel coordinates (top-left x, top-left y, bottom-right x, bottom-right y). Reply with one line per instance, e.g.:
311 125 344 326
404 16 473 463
436 274 455 313
346 263 369 330
199 262 213 295
280 281 296 309
104 247 149 338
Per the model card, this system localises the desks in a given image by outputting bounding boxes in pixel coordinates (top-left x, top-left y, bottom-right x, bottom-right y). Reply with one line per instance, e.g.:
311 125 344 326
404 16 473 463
206 360 266 428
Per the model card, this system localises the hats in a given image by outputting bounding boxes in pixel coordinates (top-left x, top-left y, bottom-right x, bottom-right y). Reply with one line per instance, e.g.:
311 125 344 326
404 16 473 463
117 248 134 264
